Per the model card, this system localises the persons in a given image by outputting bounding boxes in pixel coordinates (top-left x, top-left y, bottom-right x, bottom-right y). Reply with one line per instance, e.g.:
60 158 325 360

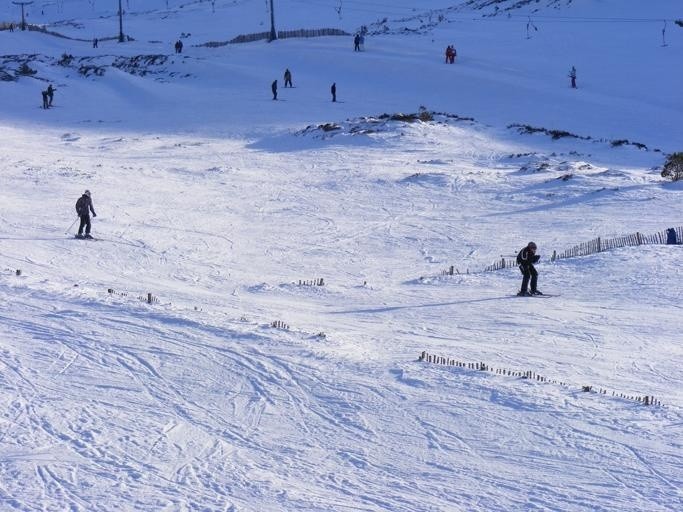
331 82 336 102
272 80 277 100
175 39 183 53
9 24 14 32
48 84 57 105
354 34 360 51
75 190 96 236
42 90 49 109
516 242 543 296
568 65 577 88
93 38 98 48
451 44 456 63
284 68 292 88
445 45 452 64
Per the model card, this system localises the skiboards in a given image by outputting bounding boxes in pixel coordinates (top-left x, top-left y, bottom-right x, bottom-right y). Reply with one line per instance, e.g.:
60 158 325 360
506 293 561 298
66 235 104 242
